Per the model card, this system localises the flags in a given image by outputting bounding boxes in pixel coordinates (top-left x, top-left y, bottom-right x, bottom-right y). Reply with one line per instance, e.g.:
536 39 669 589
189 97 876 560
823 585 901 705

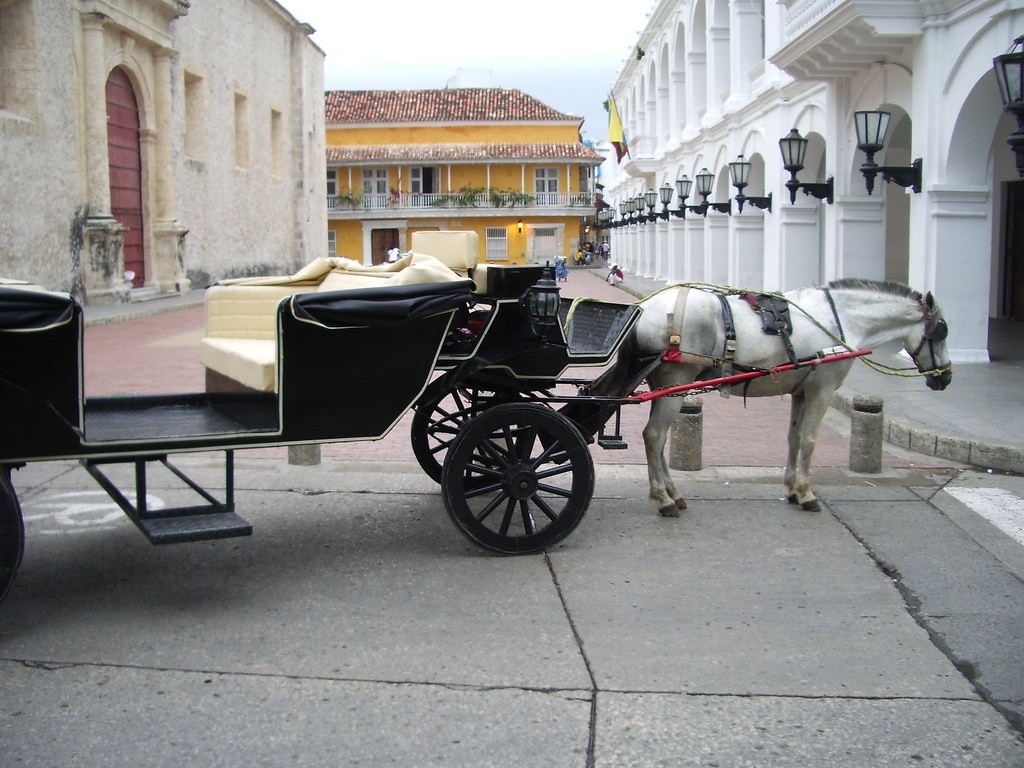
608 97 628 164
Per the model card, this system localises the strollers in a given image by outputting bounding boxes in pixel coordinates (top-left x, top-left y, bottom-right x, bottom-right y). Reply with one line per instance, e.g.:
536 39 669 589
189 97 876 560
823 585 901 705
555 256 567 281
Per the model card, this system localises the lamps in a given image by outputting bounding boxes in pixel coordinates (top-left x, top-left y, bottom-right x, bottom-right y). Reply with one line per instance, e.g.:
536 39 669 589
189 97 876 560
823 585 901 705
597 167 731 230
779 128 834 204
854 111 922 196
729 153 772 214
518 219 523 233
992 34 1024 178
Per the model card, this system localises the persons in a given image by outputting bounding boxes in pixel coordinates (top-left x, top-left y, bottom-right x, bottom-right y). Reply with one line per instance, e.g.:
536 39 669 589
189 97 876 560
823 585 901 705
388 245 400 263
606 263 623 286
576 240 609 267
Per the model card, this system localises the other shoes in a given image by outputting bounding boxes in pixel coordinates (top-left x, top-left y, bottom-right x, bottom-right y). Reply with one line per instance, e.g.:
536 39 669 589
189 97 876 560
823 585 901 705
609 284 615 286
583 265 585 266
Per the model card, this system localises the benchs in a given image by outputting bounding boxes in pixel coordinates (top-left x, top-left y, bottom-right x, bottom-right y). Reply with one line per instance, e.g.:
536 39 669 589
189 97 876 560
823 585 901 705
194 229 556 393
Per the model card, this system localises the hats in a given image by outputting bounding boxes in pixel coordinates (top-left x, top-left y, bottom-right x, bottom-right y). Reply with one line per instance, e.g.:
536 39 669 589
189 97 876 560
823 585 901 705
603 240 607 242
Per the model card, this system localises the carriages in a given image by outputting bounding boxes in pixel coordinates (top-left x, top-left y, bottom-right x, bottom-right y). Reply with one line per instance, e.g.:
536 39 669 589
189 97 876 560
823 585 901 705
0 262 953 604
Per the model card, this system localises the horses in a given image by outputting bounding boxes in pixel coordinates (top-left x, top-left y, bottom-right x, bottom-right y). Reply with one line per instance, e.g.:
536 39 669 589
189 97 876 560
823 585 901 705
617 277 953 518
